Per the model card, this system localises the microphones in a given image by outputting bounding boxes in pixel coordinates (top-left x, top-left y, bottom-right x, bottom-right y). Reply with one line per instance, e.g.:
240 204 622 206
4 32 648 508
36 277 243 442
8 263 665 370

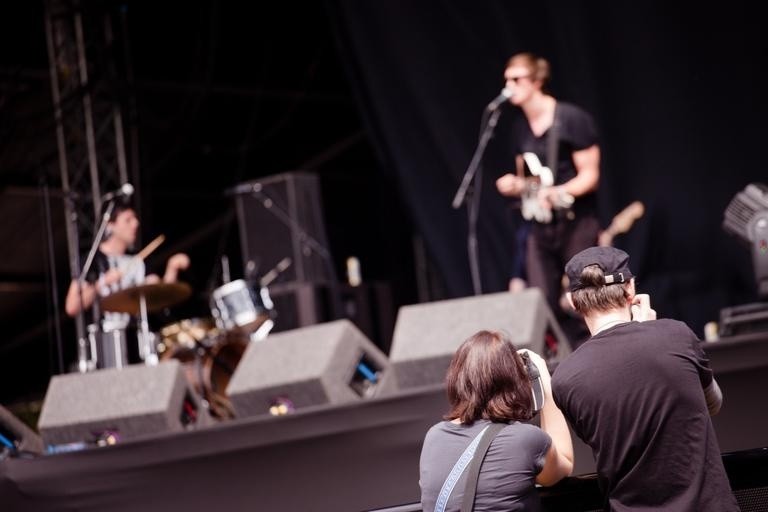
488 88 513 112
99 182 135 200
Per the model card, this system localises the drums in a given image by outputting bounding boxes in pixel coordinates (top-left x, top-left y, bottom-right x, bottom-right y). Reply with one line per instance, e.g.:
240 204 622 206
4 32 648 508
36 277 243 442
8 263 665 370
210 280 277 336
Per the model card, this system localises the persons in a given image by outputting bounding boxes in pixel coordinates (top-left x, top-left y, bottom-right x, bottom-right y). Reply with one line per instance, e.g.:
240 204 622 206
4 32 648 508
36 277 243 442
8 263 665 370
493 50 607 350
62 196 191 361
551 244 744 512
418 330 576 512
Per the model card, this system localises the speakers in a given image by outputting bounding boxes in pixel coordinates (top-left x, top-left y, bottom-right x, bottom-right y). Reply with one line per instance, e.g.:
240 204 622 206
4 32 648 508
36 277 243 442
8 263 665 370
231 171 336 285
224 317 389 414
375 287 572 392
35 357 208 446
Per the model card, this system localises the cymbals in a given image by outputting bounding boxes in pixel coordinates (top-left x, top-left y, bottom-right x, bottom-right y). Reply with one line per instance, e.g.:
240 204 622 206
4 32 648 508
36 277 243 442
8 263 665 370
101 284 191 311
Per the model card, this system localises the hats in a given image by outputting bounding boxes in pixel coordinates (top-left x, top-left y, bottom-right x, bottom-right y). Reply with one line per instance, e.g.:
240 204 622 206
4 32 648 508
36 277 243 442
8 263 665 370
565 247 633 291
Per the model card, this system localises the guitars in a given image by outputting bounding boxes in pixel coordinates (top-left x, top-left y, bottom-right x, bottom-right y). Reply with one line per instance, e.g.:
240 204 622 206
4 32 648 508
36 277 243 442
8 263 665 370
519 152 575 223
